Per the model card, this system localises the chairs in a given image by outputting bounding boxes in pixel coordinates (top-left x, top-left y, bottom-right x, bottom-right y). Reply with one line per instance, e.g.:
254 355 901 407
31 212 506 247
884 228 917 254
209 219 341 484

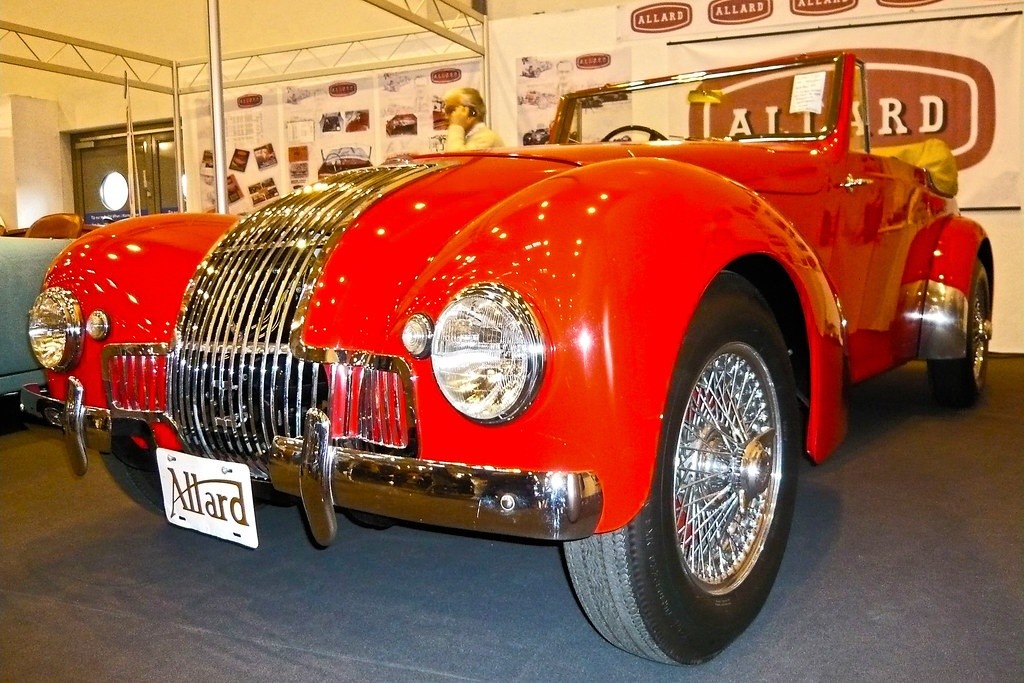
22 213 84 240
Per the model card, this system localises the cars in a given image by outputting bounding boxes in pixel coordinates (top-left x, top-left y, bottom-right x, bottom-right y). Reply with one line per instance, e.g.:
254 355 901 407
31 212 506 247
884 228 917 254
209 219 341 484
0 213 103 395
22 48 997 668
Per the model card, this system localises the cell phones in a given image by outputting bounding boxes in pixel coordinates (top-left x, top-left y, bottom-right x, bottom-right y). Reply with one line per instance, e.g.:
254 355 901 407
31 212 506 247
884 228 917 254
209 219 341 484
468 110 478 117
